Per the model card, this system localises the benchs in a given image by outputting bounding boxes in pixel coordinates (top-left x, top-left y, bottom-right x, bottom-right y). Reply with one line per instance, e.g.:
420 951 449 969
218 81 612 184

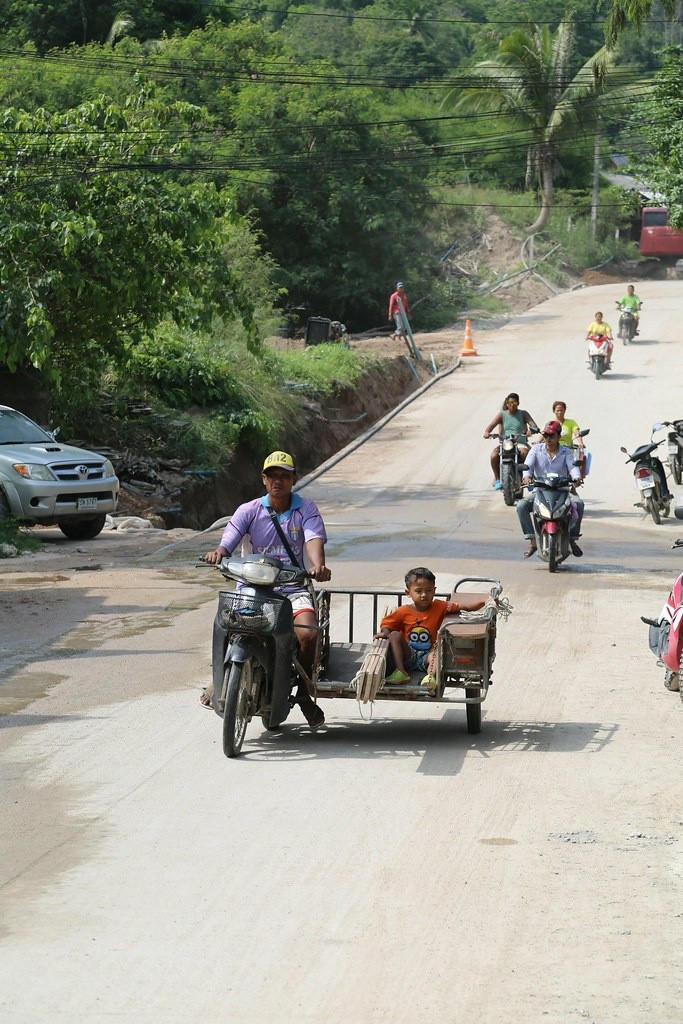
437 577 501 640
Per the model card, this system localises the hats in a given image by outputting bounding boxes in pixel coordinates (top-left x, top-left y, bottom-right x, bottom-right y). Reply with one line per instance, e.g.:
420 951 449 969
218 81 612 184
396 282 404 288
262 451 295 473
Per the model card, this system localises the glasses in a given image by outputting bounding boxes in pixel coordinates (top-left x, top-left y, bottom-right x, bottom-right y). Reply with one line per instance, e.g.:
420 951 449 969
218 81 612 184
507 401 518 406
264 471 291 479
542 433 556 439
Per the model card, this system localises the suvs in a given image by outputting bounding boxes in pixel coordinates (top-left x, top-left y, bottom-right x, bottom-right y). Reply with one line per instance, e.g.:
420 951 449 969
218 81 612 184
0 404 120 540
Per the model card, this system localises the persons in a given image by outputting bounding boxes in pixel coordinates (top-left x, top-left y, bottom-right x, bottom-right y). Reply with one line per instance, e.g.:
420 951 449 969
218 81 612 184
388 282 411 341
516 421 584 559
616 285 641 337
585 312 613 369
483 393 537 490
198 451 332 729
532 402 583 449
373 567 499 686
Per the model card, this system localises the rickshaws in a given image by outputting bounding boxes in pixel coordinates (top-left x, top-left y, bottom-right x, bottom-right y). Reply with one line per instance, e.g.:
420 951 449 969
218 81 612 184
195 554 503 757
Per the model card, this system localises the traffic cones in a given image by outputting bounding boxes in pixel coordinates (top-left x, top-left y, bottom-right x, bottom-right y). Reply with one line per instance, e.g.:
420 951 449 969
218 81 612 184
461 319 477 357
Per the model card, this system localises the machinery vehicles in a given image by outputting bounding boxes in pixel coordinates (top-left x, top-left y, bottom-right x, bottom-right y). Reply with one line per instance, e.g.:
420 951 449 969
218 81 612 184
637 206 683 280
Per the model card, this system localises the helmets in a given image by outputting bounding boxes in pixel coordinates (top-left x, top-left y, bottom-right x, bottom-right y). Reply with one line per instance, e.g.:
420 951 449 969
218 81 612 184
542 421 562 435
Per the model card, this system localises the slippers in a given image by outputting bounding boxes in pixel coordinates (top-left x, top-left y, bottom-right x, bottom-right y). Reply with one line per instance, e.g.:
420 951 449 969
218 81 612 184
199 686 215 709
300 697 324 728
418 674 437 689
386 668 410 684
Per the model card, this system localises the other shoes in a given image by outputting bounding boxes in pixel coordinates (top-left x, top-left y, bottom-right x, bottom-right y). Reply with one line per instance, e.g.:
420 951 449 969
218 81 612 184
571 545 583 557
494 479 500 490
608 361 614 370
586 361 591 369
517 471 523 478
617 331 621 335
635 332 639 335
524 546 537 558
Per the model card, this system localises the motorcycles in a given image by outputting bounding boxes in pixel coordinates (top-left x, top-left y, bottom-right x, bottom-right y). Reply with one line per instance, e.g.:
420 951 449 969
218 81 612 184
487 431 528 506
516 460 585 571
530 425 589 450
587 333 610 380
667 419 683 486
614 301 644 346
621 423 673 526
